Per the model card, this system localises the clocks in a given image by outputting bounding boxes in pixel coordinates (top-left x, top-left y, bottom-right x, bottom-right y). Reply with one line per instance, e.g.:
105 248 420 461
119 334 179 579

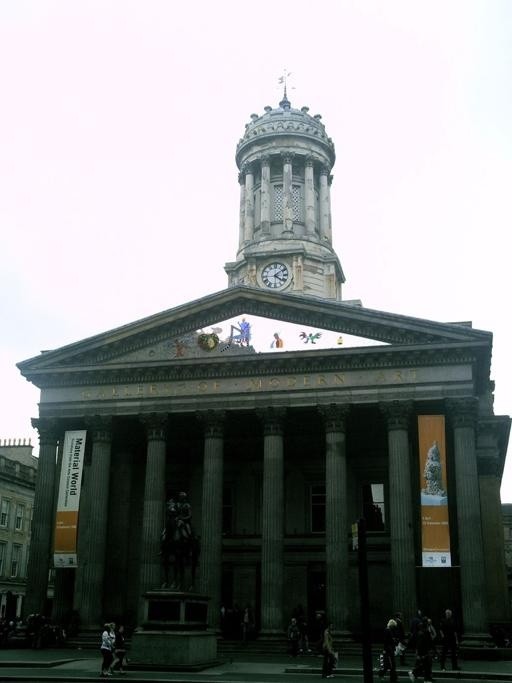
256 256 294 292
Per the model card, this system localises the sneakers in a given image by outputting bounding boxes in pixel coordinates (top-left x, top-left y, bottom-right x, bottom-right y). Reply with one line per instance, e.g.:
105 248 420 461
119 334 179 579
377 671 432 683
326 674 337 679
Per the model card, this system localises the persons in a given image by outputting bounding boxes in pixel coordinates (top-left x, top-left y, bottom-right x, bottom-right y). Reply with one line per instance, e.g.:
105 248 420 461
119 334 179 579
218 603 461 683
99 621 127 677
156 491 194 557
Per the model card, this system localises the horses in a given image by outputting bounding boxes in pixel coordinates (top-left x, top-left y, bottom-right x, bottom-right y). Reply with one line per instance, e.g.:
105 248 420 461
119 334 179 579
160 497 201 588
